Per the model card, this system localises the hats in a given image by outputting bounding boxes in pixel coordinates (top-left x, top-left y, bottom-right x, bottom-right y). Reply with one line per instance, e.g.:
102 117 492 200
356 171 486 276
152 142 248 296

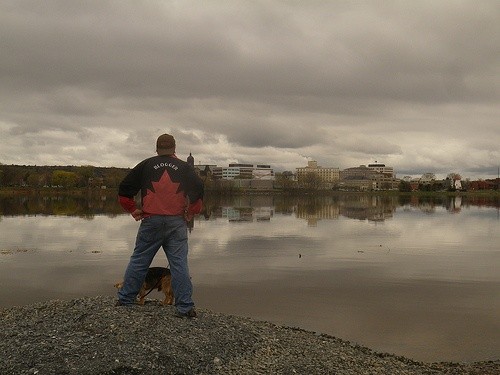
156 134 175 156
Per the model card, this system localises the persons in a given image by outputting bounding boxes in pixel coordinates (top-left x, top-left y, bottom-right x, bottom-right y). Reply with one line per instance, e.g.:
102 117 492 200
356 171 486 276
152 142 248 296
117 133 206 318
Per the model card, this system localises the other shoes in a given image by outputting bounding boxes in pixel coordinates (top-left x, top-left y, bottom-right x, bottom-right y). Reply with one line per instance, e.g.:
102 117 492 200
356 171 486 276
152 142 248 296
116 301 124 307
178 309 196 317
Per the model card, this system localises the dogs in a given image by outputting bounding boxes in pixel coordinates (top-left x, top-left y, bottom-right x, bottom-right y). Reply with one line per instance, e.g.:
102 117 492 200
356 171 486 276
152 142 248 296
115 267 192 305
